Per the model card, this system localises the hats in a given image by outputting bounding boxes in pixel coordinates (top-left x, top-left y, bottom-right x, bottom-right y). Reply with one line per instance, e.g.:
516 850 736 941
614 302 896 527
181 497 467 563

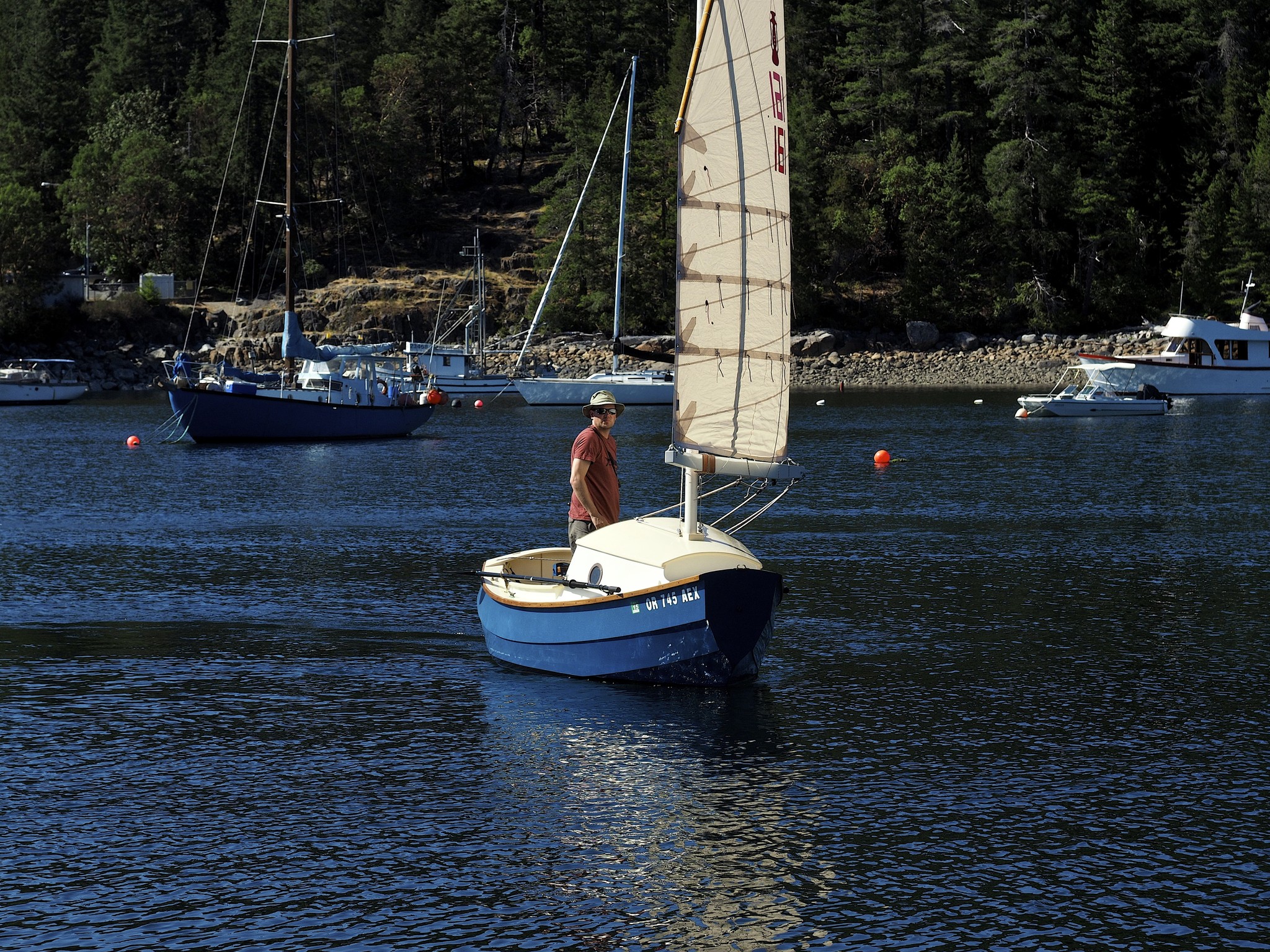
582 390 625 419
198 343 215 354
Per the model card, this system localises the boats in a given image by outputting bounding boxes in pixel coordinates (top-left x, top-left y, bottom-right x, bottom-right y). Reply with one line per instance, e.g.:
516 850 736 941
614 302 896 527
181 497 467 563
154 1 435 442
1 354 88 405
513 53 679 413
1017 363 1171 420
309 230 520 406
1082 294 1267 396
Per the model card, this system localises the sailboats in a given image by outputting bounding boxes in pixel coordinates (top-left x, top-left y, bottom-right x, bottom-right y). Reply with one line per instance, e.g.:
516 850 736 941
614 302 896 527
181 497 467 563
480 0 820 689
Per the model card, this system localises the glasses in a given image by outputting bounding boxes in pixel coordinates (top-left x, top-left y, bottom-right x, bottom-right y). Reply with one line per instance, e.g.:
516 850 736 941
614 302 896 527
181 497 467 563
590 408 617 415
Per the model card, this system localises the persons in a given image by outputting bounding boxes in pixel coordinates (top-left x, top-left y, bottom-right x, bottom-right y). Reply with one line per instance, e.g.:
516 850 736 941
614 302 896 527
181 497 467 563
567 390 628 557
